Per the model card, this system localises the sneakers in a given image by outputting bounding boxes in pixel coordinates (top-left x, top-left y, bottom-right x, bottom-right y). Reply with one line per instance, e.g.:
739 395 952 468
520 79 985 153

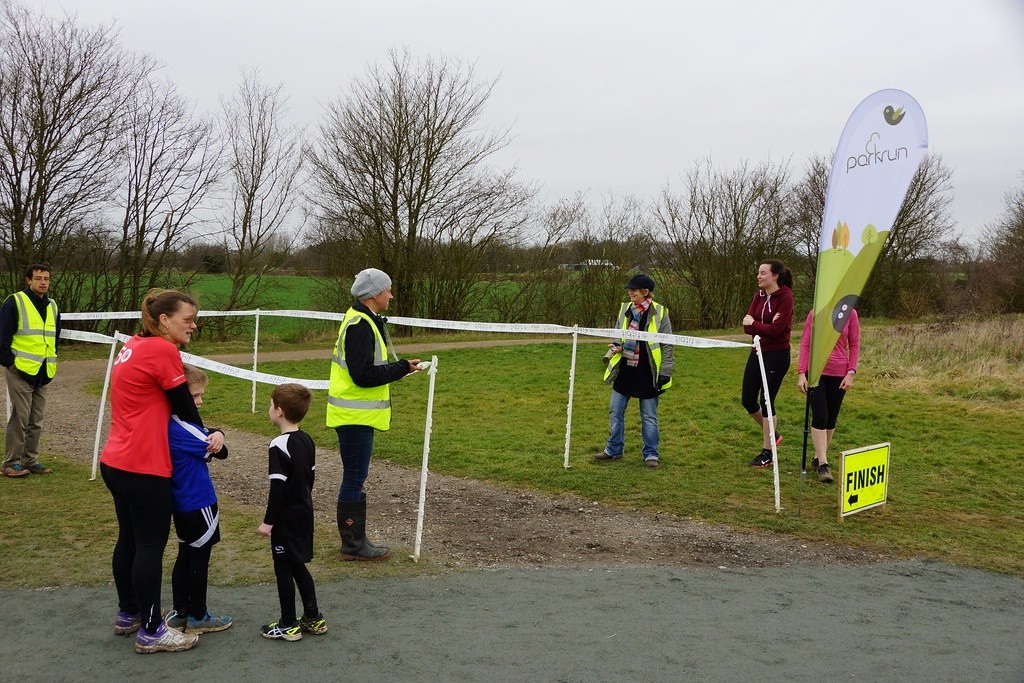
185 609 233 634
135 610 199 654
259 618 303 641
775 430 782 446
818 463 834 482
113 610 142 634
20 462 53 474
811 457 820 471
748 448 772 467
165 611 188 632
297 612 328 635
0 464 30 476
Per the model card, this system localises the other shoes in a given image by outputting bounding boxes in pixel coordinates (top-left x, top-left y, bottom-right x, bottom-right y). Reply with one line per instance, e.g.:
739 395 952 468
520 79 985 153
594 451 620 460
645 459 659 467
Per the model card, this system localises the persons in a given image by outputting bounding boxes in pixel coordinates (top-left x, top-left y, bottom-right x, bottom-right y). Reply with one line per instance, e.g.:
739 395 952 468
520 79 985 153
0 264 61 476
742 259 793 468
595 275 674 467
326 268 424 561
256 384 327 642
98 288 200 653
798 307 860 482
167 365 233 636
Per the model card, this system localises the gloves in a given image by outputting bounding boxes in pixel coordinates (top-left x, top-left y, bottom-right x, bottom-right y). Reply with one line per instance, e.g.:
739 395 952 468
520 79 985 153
655 375 671 391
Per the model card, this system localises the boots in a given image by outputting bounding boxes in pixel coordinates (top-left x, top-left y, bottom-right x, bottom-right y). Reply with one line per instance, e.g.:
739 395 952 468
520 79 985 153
361 492 392 556
337 500 390 560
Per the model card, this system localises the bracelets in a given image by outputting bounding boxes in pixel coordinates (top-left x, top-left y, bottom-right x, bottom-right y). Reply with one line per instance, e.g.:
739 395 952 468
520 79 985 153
847 371 854 375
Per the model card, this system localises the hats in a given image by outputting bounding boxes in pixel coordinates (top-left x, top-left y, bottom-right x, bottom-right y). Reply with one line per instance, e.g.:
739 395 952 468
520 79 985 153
624 275 655 292
350 268 392 300
